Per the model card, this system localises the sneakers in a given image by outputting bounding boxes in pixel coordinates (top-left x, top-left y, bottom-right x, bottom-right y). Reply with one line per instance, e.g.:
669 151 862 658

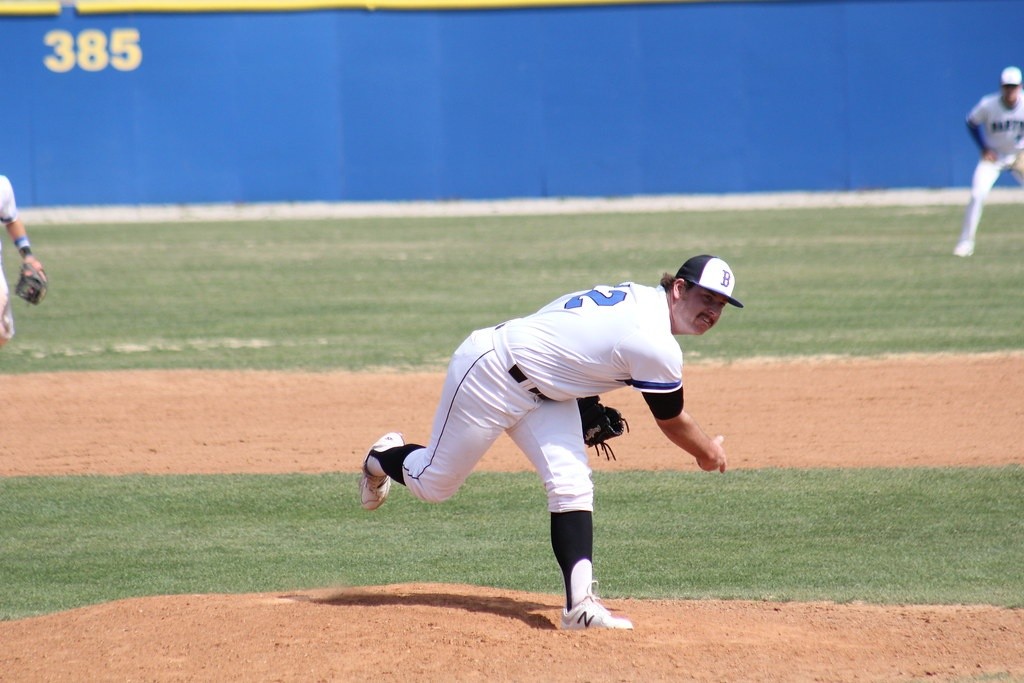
560 598 634 631
359 431 406 511
953 241 975 257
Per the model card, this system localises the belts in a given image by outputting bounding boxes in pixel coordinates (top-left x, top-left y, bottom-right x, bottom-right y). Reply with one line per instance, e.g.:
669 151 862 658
508 364 556 402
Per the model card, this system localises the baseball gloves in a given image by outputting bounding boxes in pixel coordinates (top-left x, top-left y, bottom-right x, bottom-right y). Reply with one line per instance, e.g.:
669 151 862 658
15 257 46 303
576 395 626 448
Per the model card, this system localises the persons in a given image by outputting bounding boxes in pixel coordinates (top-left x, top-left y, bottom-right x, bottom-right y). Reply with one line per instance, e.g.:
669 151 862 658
953 67 1024 257
0 172 48 349
360 255 744 631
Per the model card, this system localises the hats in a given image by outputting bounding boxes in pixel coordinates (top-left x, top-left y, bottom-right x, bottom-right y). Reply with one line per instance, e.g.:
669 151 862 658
675 254 745 308
1000 66 1022 87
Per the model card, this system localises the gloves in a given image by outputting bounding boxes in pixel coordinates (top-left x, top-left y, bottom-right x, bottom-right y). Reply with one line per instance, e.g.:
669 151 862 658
1009 151 1024 176
